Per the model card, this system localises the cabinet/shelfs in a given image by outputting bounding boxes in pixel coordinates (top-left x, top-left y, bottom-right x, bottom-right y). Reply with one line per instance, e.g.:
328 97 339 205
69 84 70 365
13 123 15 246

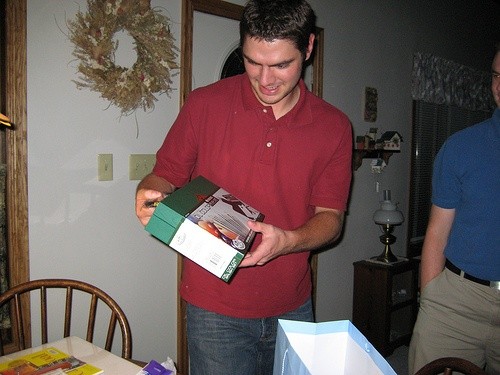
353 256 422 356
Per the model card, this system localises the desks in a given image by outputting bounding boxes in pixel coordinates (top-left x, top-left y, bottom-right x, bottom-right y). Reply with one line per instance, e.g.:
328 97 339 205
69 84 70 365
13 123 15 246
0 336 143 375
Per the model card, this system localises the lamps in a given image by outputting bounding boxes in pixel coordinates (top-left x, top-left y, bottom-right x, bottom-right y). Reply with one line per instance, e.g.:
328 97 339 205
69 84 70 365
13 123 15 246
374 190 404 263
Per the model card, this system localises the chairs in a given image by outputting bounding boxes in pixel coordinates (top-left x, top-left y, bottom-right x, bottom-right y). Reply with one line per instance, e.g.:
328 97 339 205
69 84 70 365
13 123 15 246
0 279 132 359
415 358 489 375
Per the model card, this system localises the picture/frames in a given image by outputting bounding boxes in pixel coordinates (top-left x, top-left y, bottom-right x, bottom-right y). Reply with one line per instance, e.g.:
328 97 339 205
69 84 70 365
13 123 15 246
180 0 324 112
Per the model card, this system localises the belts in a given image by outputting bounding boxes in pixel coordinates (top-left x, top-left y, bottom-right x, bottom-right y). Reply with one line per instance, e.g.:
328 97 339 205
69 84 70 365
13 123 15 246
444 258 500 291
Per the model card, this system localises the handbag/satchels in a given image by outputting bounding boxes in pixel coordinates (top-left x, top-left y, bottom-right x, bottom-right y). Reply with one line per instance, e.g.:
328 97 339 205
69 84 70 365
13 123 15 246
272 319 398 375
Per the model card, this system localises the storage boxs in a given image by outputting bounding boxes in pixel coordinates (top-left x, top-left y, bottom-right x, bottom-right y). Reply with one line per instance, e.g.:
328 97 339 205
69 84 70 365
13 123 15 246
145 175 265 282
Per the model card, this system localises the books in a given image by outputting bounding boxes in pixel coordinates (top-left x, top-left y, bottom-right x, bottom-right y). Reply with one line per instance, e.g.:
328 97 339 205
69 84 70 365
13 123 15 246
0 346 104 375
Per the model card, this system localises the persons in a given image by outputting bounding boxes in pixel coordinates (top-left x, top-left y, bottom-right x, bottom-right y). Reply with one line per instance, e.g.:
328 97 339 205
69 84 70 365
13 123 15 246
136 0 354 375
407 50 500 375
